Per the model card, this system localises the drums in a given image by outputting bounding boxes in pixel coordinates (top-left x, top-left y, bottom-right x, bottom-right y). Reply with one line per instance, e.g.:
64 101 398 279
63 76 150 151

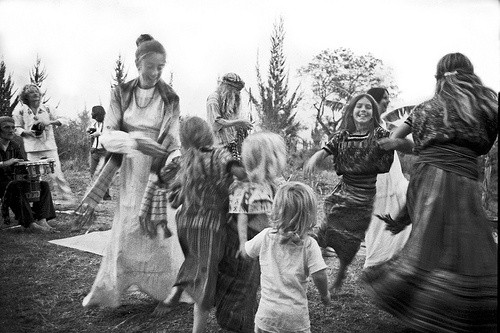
15 158 56 202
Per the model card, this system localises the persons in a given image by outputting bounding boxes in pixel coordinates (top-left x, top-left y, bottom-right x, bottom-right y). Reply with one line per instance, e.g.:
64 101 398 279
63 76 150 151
0 84 71 232
82 33 500 333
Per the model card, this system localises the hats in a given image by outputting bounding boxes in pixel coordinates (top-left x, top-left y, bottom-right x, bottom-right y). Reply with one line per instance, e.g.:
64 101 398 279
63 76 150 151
217 72 246 91
